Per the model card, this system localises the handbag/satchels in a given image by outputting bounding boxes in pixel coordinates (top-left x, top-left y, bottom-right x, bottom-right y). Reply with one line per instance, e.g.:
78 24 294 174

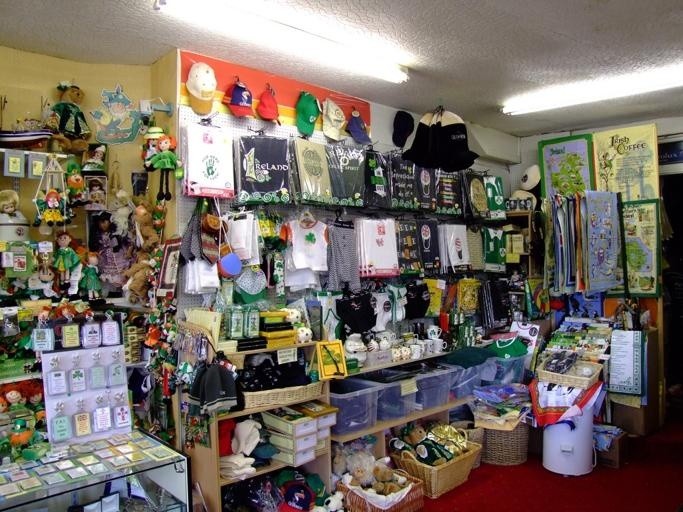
203 214 220 233
242 359 280 390
219 253 241 278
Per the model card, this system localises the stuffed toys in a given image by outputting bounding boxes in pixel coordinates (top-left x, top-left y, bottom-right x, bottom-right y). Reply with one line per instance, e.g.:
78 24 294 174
43 83 92 151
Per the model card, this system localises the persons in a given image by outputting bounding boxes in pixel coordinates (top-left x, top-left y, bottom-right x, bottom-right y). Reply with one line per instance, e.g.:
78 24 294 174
3 128 182 460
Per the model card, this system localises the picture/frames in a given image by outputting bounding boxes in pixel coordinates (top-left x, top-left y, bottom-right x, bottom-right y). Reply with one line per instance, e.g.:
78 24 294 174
538 133 596 199
604 199 660 298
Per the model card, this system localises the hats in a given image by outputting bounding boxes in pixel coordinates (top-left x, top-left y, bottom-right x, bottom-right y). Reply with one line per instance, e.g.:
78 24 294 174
186 63 216 114
222 81 256 119
274 466 331 512
232 413 280 466
322 97 345 142
256 90 279 120
190 364 237 414
297 92 319 137
393 111 414 147
402 111 479 172
346 109 371 144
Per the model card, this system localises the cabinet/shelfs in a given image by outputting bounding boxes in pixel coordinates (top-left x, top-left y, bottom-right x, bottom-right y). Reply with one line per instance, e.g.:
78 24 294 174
323 351 485 488
1 428 191 512
171 320 332 512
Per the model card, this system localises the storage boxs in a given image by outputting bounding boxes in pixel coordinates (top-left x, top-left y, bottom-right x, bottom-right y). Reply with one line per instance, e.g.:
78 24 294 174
331 354 526 434
